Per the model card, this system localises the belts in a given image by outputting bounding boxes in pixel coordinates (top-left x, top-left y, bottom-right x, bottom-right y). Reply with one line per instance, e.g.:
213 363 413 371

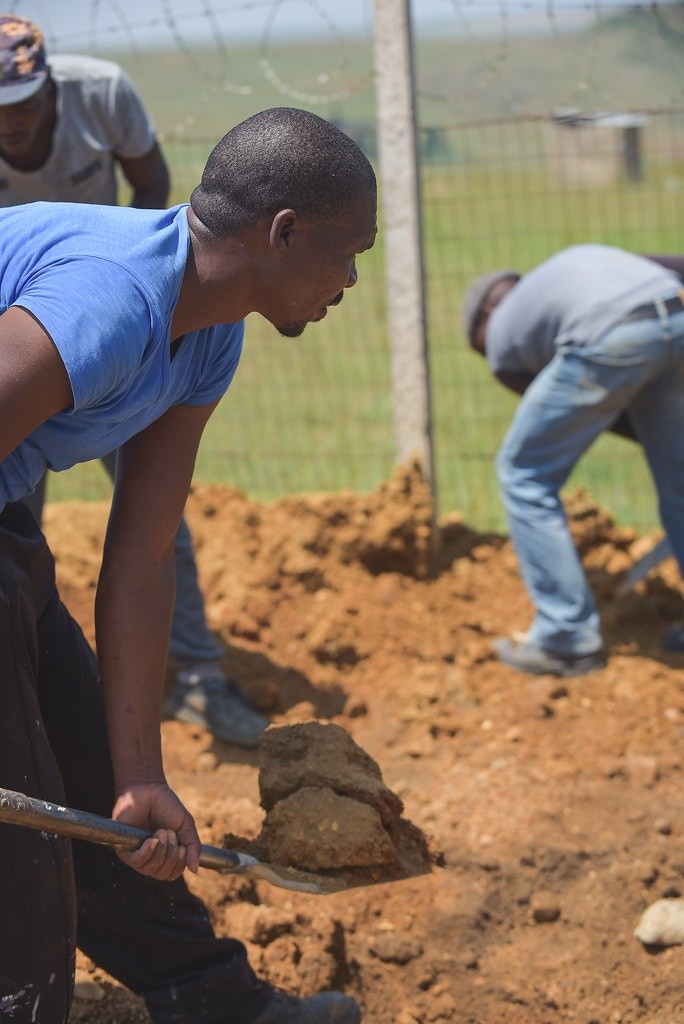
630 296 684 321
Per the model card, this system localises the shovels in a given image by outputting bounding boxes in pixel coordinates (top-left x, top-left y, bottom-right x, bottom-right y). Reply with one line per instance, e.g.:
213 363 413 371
1 787 434 898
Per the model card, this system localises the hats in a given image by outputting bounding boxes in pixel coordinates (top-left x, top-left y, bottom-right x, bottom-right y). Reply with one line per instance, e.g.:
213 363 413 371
0 14 48 106
462 272 520 348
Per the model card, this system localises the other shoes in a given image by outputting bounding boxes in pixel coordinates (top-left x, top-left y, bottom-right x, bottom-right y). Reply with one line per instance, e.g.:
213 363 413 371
665 628 684 651
495 639 606 675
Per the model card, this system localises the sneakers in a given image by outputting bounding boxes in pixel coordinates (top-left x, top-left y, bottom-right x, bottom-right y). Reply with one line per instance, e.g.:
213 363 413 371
165 678 273 748
250 986 361 1024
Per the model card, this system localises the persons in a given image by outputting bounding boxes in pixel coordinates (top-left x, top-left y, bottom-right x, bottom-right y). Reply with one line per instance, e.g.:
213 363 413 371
0 11 380 1024
462 244 684 677
